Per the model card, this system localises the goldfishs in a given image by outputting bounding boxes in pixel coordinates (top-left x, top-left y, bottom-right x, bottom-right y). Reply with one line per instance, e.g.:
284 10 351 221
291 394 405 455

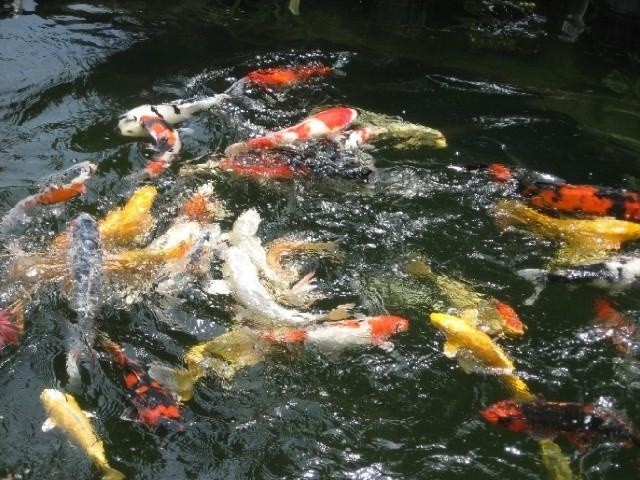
0 64 640 480
